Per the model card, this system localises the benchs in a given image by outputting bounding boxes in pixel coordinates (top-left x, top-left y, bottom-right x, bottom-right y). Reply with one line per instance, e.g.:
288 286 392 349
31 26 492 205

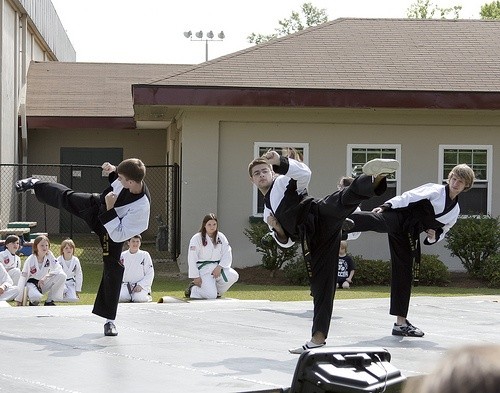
0 232 48 256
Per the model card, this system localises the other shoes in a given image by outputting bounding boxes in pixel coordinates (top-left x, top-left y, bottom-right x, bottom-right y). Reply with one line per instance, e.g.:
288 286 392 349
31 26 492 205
44 300 55 306
184 281 195 296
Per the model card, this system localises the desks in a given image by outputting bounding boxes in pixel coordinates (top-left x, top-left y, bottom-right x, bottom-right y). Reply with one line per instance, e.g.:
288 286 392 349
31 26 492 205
0 221 38 255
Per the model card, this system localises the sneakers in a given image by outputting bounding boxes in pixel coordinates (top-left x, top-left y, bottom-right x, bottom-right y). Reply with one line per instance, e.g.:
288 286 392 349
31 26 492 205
342 219 354 230
392 319 424 336
362 158 400 177
289 340 326 354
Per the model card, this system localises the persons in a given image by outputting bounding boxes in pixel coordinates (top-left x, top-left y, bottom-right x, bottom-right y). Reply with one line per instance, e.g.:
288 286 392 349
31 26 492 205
17 235 66 306
119 235 154 302
336 241 355 289
423 343 500 393
57 239 83 302
249 151 399 354
337 178 362 240
15 158 151 335
0 263 19 301
0 235 21 285
184 214 238 299
341 164 474 336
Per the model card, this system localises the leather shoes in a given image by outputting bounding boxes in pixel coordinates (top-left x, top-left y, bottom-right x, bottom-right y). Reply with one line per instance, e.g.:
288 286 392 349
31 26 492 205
104 321 118 336
15 177 35 192
